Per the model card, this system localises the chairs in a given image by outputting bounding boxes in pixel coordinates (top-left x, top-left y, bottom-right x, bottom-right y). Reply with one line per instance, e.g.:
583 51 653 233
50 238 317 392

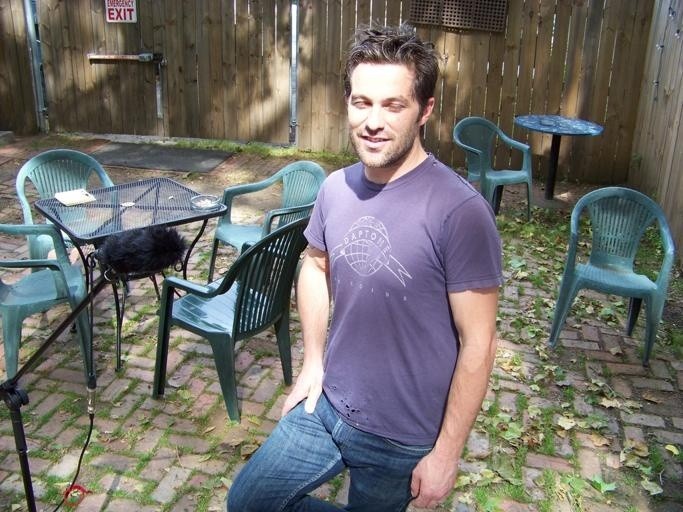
550 184 674 368
205 160 326 286
454 116 534 215
15 149 129 331
151 216 311 425
1 223 95 394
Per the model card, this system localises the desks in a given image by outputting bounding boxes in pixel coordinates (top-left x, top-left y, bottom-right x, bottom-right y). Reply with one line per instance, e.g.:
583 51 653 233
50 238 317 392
512 114 603 200
35 177 230 371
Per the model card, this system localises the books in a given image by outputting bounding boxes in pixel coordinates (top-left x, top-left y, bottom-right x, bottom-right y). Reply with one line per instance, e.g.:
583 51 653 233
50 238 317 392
55 188 96 206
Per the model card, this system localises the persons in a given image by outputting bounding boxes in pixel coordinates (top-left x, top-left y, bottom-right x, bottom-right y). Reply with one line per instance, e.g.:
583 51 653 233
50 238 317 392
226 16 504 512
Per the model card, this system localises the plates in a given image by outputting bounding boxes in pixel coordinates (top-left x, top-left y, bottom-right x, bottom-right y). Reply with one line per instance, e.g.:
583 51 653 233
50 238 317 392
190 194 221 210
